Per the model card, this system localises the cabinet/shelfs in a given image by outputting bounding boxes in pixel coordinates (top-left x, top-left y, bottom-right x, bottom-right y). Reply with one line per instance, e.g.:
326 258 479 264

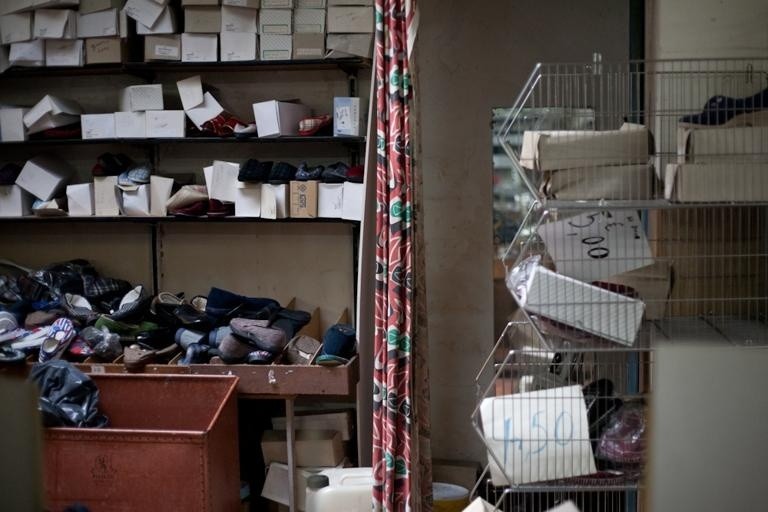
0 0 373 512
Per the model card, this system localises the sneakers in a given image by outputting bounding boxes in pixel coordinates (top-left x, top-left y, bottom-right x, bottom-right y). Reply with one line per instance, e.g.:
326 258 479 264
171 197 235 219
94 153 150 190
237 158 364 183
298 112 332 135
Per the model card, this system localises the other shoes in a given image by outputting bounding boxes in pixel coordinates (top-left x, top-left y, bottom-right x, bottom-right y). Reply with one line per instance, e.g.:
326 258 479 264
0 258 355 367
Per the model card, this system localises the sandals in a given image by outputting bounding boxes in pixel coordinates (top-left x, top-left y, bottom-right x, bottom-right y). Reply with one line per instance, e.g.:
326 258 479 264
200 112 254 136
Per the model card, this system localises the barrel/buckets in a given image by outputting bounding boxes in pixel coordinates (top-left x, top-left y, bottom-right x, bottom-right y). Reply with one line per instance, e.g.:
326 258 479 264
305 467 375 512
430 482 469 512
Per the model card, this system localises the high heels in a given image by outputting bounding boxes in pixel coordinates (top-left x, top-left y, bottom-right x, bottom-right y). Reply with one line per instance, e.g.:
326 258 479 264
677 85 767 129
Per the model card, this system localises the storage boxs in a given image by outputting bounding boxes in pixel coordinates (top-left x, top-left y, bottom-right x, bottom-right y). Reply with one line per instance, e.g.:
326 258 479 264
526 206 767 347
519 117 766 205
41 374 243 510
2 1 373 67
0 75 372 141
259 406 355 474
2 155 367 220
